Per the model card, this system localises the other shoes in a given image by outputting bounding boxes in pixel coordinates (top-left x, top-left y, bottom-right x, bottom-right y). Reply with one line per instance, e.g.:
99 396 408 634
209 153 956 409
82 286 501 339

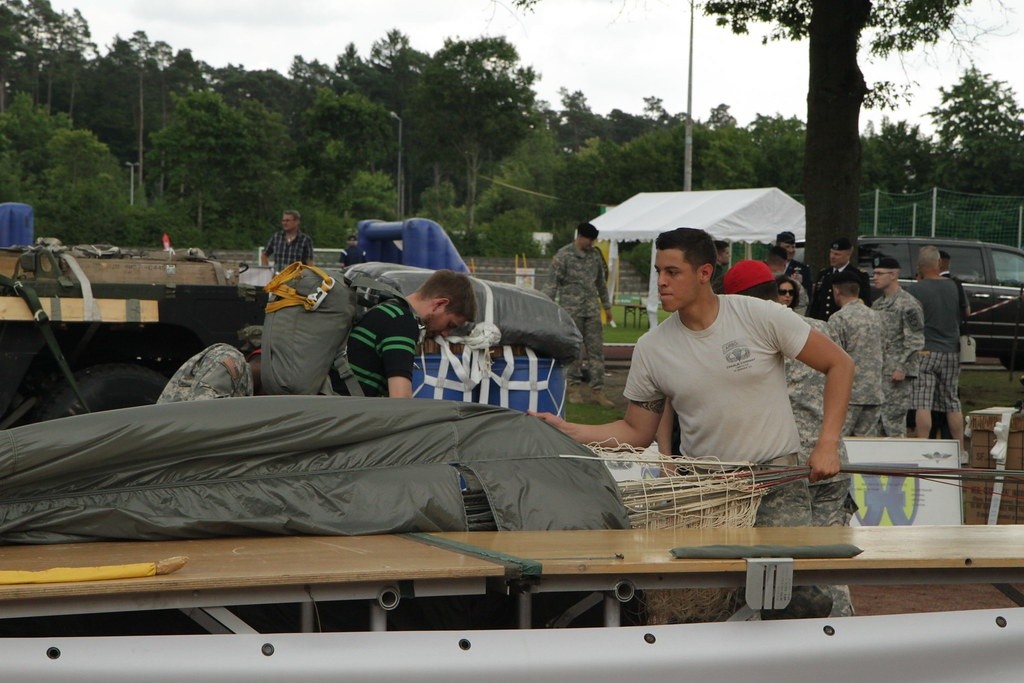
568 384 585 403
960 450 970 464
590 393 615 409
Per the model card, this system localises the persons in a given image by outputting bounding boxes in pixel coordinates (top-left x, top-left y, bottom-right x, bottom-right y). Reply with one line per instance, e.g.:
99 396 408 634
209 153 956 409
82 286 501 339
711 239 730 294
764 247 809 316
938 251 970 326
244 350 270 395
869 256 925 438
541 223 616 409
827 273 891 438
339 235 367 270
527 225 855 624
906 244 969 466
806 238 872 322
318 269 477 400
656 260 857 617
261 210 314 279
776 231 812 300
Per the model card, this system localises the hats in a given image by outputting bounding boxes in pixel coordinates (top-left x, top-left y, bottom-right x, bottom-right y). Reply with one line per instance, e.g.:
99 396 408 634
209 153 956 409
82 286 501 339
871 256 902 270
938 250 950 260
828 271 862 286
723 258 776 294
829 237 853 250
777 231 795 246
577 222 599 239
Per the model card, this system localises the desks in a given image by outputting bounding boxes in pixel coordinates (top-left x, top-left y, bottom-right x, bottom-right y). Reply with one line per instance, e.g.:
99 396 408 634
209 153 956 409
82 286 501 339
0 530 526 637
408 526 1024 629
614 303 650 329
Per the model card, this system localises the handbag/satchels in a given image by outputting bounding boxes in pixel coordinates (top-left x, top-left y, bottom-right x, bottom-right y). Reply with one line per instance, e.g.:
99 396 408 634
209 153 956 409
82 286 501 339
958 335 977 363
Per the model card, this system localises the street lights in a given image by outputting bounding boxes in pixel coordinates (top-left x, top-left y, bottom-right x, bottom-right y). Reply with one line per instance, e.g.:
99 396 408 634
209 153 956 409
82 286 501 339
389 112 402 220
125 162 133 205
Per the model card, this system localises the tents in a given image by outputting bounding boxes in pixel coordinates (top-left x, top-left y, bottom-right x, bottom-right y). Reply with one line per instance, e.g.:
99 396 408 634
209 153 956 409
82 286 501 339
574 186 806 330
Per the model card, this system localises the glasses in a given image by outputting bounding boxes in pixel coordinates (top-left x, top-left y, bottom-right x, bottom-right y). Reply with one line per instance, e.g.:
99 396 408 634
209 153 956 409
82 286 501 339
779 288 795 296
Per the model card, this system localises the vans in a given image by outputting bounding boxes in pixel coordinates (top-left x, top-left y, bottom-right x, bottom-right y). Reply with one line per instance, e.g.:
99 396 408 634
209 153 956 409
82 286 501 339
793 234 1024 370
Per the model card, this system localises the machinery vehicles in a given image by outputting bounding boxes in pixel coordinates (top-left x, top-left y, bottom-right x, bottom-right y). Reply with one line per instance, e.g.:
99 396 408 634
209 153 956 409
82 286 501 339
1 246 273 428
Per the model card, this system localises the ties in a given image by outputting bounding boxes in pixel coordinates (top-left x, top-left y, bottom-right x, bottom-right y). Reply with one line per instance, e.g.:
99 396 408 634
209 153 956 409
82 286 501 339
834 269 839 274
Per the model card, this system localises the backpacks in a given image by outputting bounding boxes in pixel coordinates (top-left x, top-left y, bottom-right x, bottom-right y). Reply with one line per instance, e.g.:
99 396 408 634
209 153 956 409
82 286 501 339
259 261 423 396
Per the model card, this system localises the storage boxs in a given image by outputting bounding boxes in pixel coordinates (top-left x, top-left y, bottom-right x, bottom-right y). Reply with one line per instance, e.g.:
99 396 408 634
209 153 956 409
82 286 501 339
415 338 465 354
967 406 1024 433
413 355 565 421
965 476 1024 524
489 345 526 358
969 431 1023 470
